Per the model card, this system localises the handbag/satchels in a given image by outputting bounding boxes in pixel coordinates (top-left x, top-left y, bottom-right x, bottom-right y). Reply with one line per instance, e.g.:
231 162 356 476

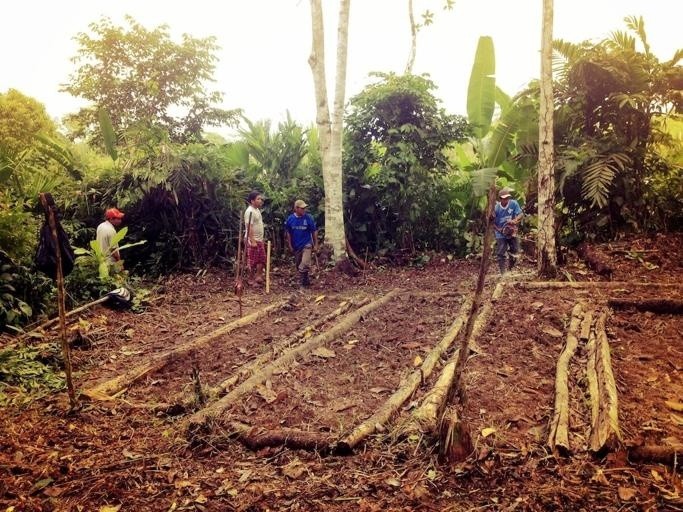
34 193 76 279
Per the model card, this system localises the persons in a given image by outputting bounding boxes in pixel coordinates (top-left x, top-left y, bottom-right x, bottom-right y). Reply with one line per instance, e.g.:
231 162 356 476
493 190 525 276
244 192 266 285
285 200 318 288
96 208 125 272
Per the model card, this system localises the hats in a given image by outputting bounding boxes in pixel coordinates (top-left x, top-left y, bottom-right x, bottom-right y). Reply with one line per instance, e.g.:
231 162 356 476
498 189 511 199
295 199 309 209
106 208 124 220
